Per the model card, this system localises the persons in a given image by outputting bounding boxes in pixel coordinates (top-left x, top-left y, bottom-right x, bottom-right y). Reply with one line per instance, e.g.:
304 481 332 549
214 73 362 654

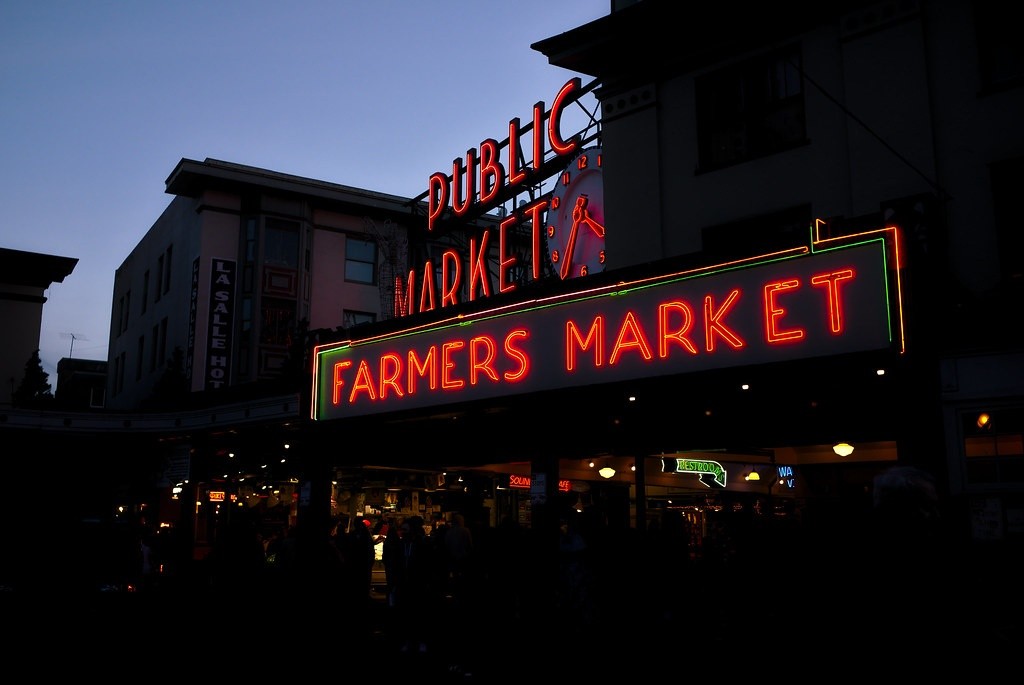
381 514 474 655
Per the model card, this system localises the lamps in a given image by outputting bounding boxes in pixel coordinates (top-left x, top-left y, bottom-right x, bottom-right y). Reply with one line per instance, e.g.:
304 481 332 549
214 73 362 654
598 466 616 479
833 442 854 457
